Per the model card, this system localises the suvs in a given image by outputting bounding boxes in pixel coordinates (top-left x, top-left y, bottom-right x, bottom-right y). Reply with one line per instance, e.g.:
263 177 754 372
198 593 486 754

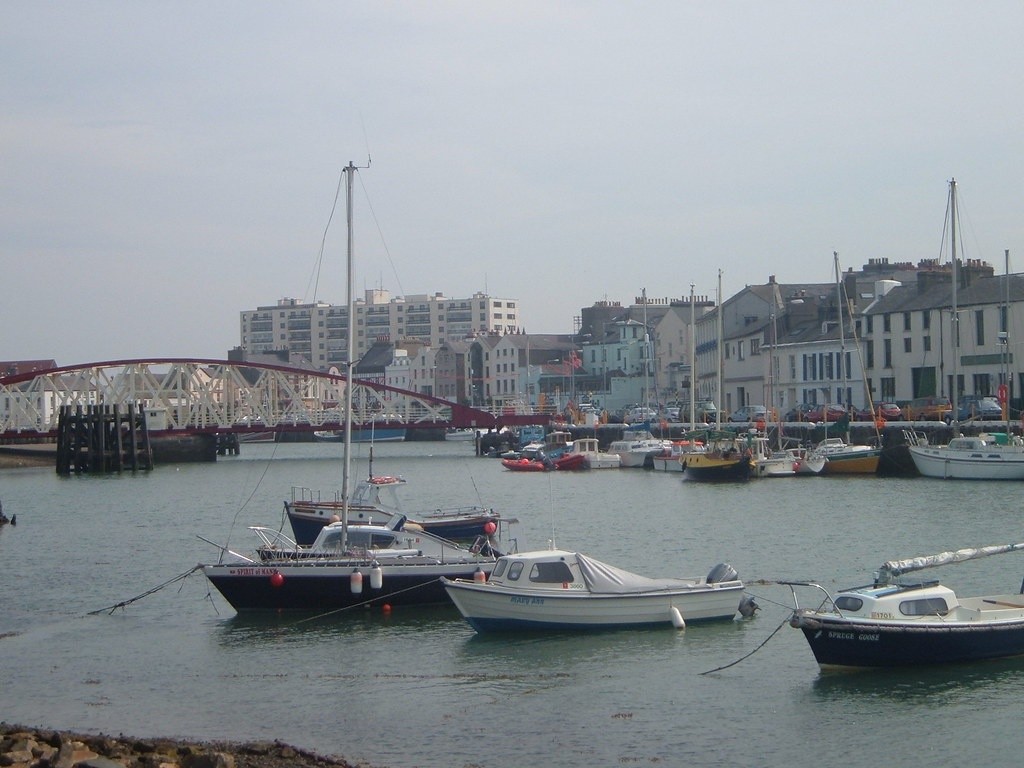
684 400 726 424
903 395 951 422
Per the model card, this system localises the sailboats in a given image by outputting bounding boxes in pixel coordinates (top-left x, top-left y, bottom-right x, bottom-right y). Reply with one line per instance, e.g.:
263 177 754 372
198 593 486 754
202 154 541 623
500 176 1024 483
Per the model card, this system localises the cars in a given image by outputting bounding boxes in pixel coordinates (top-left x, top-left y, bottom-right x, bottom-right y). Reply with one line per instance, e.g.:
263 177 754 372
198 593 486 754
854 402 903 423
584 408 604 423
783 403 819 422
801 403 847 423
943 399 1003 425
608 409 625 423
623 408 657 425
659 407 682 423
727 405 772 423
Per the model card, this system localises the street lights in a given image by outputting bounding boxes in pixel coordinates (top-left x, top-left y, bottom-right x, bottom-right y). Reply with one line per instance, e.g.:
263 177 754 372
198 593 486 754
546 358 560 399
426 365 437 396
570 333 592 403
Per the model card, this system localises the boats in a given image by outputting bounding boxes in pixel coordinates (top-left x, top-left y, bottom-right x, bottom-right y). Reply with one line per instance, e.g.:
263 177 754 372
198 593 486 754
738 539 1023 676
283 410 500 549
435 468 762 637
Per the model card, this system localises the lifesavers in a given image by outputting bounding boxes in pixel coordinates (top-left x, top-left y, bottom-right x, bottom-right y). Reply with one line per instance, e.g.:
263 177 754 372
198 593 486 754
370 477 393 483
945 415 952 426
